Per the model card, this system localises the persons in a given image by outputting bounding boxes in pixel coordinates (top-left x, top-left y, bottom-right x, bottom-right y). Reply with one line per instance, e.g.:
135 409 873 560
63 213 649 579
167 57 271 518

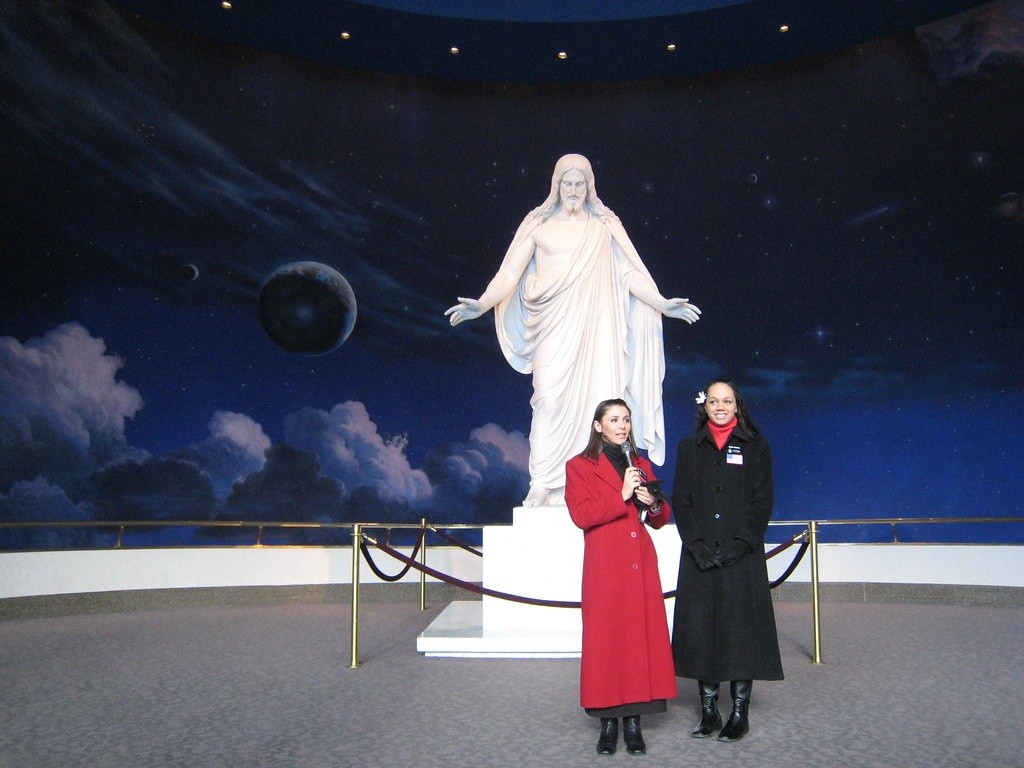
445 154 701 506
672 379 784 744
565 375 678 756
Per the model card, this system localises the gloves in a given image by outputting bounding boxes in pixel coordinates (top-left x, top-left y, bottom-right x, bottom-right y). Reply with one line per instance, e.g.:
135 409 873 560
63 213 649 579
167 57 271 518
688 539 722 571
715 538 750 567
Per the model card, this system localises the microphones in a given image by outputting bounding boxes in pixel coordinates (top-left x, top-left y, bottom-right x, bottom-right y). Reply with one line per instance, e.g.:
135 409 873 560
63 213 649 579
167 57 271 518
621 442 639 490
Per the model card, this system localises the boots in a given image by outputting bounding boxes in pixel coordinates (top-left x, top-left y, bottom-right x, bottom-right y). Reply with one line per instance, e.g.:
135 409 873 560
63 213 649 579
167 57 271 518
718 679 753 742
691 679 723 738
597 718 618 755
623 715 646 755
